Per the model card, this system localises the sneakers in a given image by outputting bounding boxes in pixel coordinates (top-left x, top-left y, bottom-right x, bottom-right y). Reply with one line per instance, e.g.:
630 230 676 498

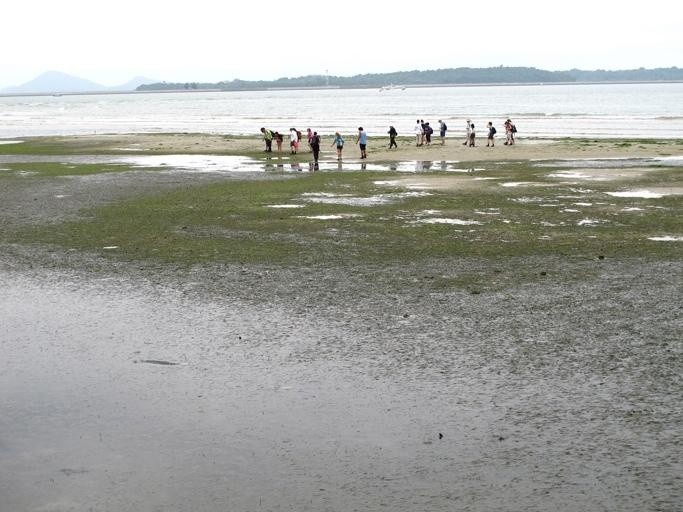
360 155 367 159
486 144 495 147
462 141 468 145
503 142 512 145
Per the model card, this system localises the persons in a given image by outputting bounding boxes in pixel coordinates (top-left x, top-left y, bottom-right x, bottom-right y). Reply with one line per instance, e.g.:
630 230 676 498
261 128 300 154
486 122 496 147
330 132 344 160
462 121 475 147
388 125 398 149
503 119 515 145
356 127 367 159
414 119 447 147
307 128 320 162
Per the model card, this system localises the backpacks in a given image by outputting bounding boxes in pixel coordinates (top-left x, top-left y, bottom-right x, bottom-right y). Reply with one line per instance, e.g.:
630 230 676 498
512 125 517 132
492 127 496 134
444 124 447 131
428 127 433 134
270 130 274 137
296 131 302 139
